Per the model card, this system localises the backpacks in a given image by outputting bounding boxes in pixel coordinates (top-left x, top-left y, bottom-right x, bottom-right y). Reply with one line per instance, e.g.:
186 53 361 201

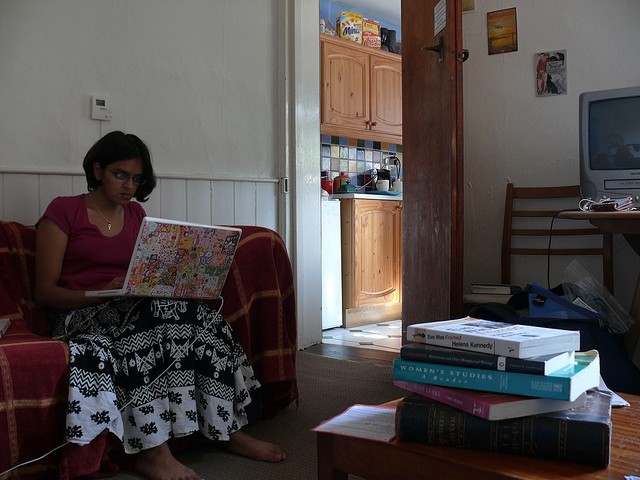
471 281 640 396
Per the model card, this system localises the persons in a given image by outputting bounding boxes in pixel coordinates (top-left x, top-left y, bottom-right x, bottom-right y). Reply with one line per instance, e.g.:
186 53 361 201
33 130 288 479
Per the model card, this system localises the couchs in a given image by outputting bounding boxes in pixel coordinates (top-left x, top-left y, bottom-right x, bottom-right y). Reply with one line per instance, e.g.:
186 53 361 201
0 221 299 480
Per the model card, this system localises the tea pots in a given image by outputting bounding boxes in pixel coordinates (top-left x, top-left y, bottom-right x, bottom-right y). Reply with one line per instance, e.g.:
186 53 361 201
384 156 402 183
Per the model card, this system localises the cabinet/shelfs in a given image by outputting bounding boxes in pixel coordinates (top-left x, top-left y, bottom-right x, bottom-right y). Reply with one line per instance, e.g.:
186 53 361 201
319 32 403 145
339 198 401 326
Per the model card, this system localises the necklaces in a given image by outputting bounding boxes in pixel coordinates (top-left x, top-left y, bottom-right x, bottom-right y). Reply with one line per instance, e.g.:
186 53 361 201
89 192 121 231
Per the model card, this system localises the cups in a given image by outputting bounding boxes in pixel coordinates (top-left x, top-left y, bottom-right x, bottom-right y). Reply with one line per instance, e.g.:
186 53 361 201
322 179 334 194
375 179 390 192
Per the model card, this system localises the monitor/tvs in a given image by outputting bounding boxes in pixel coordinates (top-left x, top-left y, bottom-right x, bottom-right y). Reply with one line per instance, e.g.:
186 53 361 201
578 85 640 210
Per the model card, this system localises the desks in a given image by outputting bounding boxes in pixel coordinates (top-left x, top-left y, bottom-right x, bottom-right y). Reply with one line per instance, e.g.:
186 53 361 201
557 210 640 361
310 386 639 480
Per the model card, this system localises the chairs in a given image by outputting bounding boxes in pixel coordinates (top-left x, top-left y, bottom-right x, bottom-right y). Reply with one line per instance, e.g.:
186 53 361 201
500 183 613 296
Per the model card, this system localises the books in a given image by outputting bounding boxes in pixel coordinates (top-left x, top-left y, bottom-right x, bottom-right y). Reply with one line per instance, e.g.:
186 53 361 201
405 314 581 360
392 380 590 422
469 283 523 294
393 389 615 469
399 341 577 376
391 350 601 401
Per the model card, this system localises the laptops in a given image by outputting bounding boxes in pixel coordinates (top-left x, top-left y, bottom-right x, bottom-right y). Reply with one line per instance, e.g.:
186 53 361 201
84 216 243 300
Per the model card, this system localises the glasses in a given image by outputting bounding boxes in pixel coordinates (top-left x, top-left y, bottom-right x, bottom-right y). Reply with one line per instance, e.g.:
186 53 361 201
106 167 146 185
